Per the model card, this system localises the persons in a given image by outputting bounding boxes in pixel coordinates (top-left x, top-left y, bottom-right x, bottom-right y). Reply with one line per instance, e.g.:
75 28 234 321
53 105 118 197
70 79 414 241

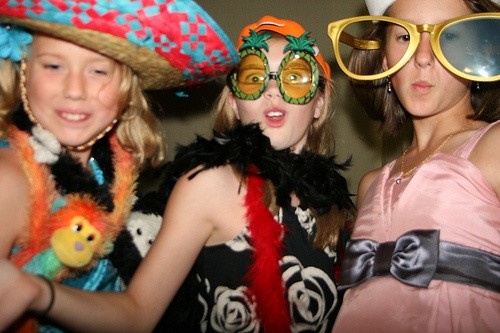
0 0 150 332
0 15 353 333
330 0 499 333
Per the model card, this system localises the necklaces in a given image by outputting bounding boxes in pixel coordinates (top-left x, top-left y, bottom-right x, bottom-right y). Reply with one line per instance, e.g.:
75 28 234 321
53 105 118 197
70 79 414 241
395 122 470 185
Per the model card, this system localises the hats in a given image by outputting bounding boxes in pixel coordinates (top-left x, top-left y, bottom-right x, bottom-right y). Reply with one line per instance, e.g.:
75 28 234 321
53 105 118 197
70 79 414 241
235 14 330 83
0 0 243 92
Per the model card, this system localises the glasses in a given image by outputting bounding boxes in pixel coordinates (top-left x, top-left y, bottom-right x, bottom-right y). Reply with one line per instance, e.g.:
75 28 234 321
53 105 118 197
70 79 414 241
327 13 500 83
226 28 326 105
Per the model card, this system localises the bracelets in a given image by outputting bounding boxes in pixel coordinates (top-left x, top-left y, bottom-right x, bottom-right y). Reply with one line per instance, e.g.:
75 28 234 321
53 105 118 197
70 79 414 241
34 274 58 315
